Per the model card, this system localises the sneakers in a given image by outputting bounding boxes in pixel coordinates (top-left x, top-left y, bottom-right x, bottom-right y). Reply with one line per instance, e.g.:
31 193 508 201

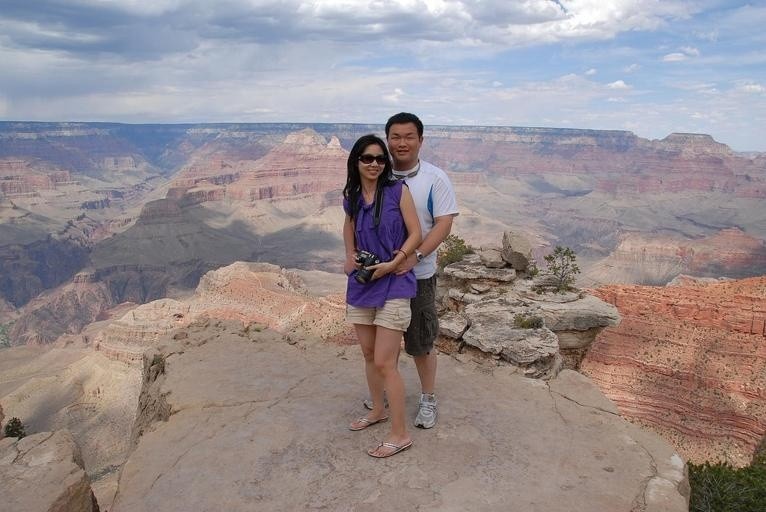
364 389 389 410
414 392 439 429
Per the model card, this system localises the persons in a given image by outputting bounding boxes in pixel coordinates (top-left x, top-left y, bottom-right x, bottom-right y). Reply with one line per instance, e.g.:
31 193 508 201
354 111 461 431
339 134 423 458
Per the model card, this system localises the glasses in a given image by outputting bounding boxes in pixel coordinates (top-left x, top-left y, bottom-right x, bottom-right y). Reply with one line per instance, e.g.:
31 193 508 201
392 171 417 179
359 153 386 165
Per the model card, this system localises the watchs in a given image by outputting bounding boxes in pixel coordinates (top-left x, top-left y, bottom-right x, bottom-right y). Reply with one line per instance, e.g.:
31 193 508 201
414 248 424 262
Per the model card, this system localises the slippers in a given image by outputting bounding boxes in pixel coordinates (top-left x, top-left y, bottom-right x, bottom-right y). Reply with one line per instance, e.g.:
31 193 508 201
348 413 388 431
367 438 413 458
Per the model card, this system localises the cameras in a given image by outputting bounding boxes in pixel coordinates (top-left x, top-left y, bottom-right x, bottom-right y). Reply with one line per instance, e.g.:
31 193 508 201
353 250 382 286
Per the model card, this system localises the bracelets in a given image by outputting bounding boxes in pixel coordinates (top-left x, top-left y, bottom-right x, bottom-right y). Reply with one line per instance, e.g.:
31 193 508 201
398 248 408 259
